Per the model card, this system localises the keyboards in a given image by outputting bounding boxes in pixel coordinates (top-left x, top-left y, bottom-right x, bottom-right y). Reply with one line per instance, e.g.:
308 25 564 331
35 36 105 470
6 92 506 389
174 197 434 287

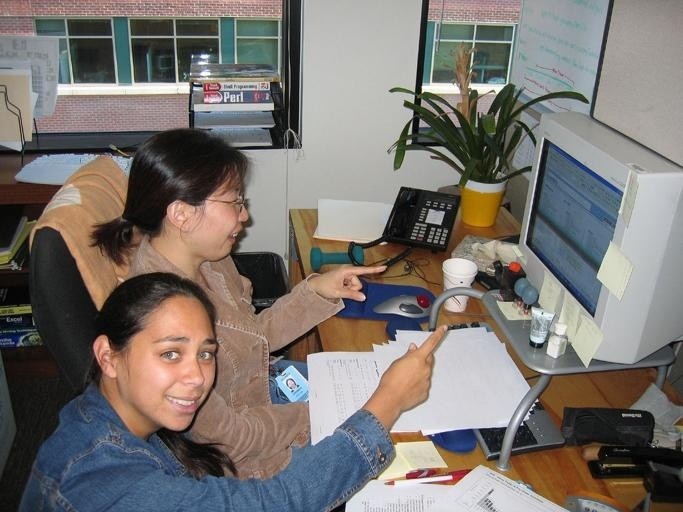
429 319 566 462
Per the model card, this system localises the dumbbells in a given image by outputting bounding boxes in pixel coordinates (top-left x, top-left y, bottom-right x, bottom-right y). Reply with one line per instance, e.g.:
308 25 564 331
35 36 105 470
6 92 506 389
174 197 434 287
310 244 365 271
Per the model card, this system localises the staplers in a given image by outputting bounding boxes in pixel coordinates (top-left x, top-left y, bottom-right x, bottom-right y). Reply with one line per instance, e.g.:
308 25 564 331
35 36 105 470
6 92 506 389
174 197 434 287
582 446 683 479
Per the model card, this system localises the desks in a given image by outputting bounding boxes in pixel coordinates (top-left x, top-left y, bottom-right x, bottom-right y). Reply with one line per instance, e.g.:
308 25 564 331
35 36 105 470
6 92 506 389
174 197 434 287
283 203 682 511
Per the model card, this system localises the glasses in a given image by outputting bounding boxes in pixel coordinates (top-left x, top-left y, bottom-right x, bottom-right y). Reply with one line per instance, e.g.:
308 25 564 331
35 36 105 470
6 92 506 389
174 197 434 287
206 193 245 212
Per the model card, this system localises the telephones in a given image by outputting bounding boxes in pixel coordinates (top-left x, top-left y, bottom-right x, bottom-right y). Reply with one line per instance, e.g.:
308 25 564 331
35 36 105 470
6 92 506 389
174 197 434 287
383 186 460 252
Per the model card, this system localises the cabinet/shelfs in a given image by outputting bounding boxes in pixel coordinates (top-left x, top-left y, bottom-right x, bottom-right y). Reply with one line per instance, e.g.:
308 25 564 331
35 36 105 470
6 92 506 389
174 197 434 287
0 153 58 362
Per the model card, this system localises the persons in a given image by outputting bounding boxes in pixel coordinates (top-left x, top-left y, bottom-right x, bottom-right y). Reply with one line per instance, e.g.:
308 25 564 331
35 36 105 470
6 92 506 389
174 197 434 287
91 129 387 478
18 273 448 511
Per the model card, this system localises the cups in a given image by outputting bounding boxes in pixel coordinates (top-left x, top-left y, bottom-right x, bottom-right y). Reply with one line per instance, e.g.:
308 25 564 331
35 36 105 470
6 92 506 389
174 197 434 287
441 258 479 315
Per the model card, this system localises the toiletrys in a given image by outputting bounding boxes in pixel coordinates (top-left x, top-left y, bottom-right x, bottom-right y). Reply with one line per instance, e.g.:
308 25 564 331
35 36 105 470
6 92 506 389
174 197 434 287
529 305 567 359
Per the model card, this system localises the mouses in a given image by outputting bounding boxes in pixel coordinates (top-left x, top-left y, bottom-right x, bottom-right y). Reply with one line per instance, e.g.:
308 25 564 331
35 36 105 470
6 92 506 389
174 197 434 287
371 293 431 319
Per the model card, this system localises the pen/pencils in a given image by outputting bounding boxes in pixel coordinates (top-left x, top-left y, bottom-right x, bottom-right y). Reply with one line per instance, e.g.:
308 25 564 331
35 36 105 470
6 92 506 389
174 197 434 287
384 469 472 485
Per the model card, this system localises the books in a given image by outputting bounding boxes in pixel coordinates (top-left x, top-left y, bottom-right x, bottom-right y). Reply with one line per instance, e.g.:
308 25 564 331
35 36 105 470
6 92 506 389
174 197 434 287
189 64 279 147
0 215 44 347
0 34 59 153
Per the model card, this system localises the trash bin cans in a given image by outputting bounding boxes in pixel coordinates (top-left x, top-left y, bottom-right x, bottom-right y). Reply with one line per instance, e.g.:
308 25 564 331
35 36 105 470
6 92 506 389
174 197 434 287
232 252 289 359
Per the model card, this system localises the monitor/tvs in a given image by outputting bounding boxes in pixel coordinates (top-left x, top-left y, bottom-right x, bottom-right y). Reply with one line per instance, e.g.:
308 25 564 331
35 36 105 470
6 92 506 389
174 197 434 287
155 54 173 69
509 111 682 366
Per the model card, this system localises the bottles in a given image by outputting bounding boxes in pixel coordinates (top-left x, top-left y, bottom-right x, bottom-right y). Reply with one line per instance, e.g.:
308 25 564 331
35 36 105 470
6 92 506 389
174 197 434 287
545 323 568 357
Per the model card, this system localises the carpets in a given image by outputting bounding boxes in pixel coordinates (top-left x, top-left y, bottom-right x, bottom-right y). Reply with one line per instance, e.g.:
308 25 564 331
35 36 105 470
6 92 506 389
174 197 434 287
0 376 74 512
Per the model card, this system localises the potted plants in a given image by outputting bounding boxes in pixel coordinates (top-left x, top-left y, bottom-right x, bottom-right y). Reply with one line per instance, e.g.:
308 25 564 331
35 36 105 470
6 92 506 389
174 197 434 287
381 35 593 230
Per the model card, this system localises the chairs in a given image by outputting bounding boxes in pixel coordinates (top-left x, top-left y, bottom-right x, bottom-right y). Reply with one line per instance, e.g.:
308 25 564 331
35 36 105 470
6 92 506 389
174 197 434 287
32 154 293 399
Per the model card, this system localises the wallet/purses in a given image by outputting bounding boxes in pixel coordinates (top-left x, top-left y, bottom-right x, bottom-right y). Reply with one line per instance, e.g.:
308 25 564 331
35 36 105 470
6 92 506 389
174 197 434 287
560 407 654 444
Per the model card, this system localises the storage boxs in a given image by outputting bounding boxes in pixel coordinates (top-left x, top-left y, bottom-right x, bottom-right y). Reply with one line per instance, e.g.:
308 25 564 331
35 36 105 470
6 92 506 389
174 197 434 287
0 299 45 349
